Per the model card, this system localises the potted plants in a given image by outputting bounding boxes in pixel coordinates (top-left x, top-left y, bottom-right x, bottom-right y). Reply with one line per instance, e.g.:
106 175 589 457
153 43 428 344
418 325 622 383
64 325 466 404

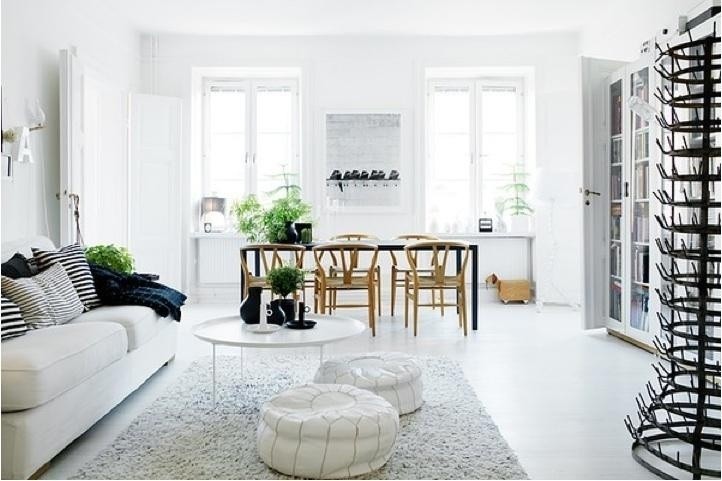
500 160 534 234
268 185 313 244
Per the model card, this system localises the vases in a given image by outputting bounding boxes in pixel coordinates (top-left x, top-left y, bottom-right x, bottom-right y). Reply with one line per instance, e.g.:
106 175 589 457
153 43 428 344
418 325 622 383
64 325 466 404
295 223 312 243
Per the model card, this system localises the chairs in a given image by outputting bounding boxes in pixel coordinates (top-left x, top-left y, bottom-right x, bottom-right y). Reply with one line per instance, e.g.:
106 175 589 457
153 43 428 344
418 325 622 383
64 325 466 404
239 235 478 336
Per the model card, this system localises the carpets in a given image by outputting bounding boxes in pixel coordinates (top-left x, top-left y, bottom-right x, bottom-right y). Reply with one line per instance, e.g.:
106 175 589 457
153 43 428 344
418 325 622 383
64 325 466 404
69 356 529 480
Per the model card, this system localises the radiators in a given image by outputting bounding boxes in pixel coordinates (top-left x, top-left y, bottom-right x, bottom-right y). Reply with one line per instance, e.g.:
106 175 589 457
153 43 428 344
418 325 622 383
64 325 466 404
198 237 291 284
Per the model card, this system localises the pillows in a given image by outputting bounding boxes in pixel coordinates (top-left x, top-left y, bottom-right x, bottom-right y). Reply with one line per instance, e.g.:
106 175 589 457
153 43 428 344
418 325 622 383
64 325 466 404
1 241 101 341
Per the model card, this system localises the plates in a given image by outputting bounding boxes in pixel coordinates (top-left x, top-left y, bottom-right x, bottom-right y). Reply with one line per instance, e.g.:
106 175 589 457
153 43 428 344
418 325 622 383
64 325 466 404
286 320 316 329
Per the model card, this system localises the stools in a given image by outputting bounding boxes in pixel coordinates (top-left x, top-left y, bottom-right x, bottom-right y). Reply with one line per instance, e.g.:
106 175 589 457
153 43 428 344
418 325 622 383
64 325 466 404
313 350 423 415
256 384 399 480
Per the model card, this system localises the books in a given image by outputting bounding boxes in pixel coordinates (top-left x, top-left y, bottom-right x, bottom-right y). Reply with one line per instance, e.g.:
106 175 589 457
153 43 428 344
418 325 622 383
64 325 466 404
610 85 649 332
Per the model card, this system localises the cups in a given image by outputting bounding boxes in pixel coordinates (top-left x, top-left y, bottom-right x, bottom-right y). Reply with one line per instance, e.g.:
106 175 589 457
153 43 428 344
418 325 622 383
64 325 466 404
205 223 211 233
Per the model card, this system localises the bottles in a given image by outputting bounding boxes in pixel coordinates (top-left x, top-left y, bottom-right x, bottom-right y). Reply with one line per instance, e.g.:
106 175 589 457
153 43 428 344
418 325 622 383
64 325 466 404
240 286 262 324
267 301 285 326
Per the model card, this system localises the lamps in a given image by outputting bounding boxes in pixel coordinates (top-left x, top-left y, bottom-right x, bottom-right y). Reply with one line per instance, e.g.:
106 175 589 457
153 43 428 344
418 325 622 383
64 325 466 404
24 98 48 132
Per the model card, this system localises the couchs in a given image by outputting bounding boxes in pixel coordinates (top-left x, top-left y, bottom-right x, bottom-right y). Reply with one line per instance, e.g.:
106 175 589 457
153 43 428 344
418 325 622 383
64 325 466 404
1 236 177 480
